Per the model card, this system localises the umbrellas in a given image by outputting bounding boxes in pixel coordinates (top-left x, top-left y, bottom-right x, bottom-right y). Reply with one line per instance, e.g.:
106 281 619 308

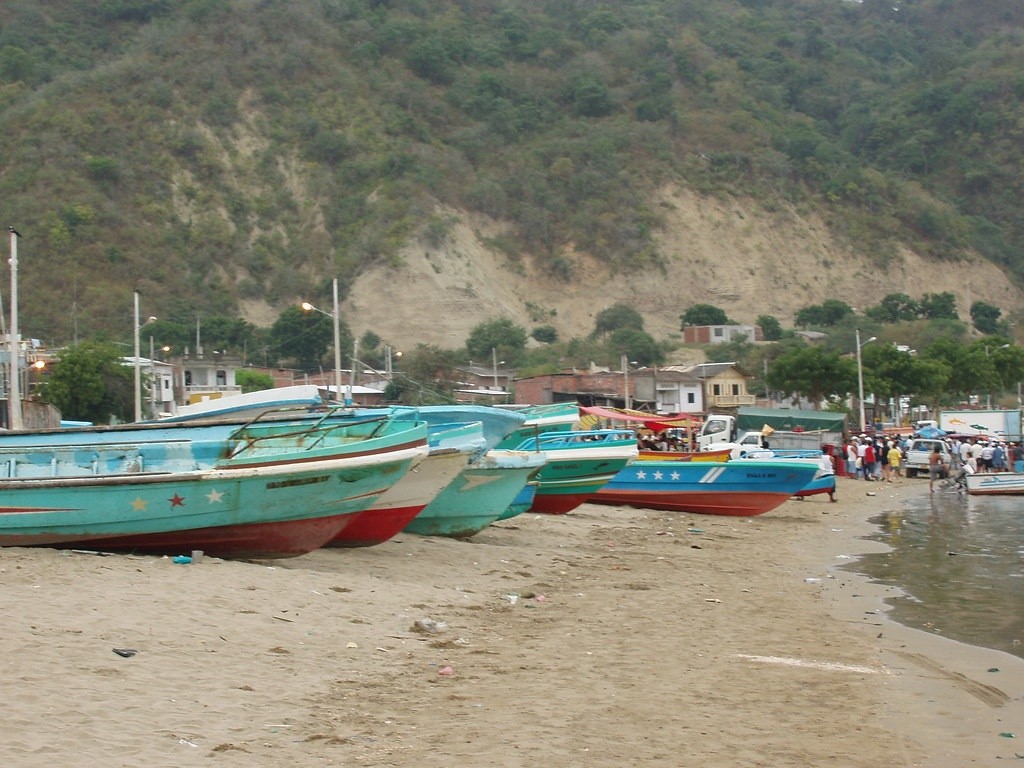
915 425 947 439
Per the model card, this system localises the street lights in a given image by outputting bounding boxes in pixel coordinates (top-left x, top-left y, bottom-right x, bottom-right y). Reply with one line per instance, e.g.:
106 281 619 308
387 345 403 379
149 335 170 414
301 278 344 401
630 360 657 414
491 347 506 387
854 328 877 434
133 291 158 423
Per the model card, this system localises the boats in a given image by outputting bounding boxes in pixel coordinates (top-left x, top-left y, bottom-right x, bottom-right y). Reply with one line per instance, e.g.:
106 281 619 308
964 472 1024 495
0 400 837 559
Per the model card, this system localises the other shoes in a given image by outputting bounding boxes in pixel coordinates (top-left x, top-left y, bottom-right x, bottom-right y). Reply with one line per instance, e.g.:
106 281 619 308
848 473 858 479
865 476 871 481
888 480 892 483
871 476 879 481
880 477 886 481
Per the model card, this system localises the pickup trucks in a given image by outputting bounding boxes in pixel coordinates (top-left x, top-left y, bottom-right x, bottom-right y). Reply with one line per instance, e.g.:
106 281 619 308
727 432 836 476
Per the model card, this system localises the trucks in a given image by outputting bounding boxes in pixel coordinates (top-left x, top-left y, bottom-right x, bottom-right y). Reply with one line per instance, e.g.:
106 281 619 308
695 406 849 457
913 409 1023 446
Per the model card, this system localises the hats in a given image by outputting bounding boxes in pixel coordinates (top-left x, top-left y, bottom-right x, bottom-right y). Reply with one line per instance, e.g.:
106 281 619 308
941 436 1016 448
851 431 900 447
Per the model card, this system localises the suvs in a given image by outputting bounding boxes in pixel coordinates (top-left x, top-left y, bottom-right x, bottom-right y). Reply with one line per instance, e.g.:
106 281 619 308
905 438 954 478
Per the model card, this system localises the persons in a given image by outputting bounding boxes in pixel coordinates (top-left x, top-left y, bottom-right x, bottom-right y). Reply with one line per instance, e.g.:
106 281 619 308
821 448 839 503
952 452 978 489
887 444 903 483
581 425 697 452
843 420 1024 483
847 440 859 480
928 445 948 493
